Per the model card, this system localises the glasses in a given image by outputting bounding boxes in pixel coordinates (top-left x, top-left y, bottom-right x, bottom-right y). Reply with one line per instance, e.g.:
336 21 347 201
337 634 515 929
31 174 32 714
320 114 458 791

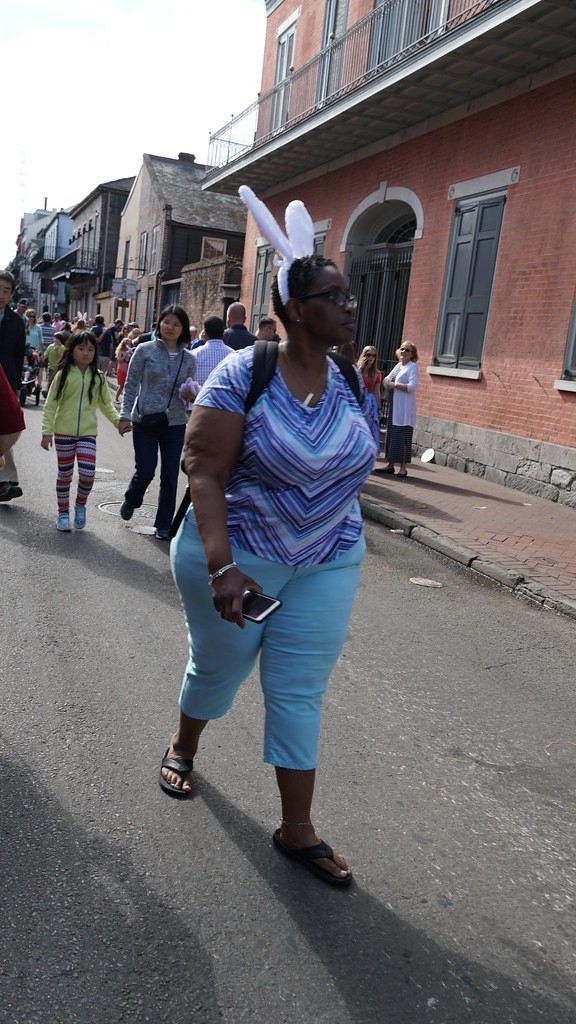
400 348 413 352
364 353 377 358
300 292 359 309
28 315 35 320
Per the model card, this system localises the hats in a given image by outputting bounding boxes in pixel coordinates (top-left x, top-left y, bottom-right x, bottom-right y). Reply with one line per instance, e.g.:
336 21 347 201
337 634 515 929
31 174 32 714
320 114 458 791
18 298 30 308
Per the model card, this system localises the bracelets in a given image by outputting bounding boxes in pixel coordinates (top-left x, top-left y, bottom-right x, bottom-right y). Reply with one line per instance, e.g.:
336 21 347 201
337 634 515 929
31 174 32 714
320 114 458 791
378 408 381 410
208 561 237 585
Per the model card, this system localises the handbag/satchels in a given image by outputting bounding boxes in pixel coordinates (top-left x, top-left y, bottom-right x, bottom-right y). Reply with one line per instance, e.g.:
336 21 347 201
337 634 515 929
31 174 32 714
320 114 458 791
140 412 170 435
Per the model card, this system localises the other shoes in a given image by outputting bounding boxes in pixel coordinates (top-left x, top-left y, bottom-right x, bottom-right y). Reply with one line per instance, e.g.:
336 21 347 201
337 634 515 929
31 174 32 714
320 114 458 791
374 467 395 474
114 400 121 404
0 482 24 503
396 471 408 478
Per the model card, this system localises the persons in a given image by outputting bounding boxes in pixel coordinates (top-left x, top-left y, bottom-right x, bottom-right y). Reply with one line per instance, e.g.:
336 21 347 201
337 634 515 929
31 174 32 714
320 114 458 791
375 341 418 478
338 341 357 367
0 269 282 539
353 346 383 420
160 257 380 892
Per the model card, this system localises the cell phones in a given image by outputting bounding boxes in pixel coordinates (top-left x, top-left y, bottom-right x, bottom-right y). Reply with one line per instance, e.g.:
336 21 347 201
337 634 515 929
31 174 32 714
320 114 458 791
242 590 283 624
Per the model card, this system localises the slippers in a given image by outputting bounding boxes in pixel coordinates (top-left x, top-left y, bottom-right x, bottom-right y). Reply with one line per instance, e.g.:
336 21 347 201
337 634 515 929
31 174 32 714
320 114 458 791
271 826 353 889
157 744 193 798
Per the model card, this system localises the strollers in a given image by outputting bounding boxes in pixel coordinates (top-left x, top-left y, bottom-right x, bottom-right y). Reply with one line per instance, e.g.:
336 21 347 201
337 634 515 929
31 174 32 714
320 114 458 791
19 343 42 406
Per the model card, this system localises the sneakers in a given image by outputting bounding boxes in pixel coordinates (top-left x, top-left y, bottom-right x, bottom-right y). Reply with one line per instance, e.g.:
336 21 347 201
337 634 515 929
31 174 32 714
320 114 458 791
155 526 171 540
73 507 87 529
120 498 136 521
55 514 72 532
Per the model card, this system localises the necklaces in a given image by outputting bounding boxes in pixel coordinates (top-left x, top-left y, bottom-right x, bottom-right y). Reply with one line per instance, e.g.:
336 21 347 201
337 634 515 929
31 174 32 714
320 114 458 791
283 346 326 405
